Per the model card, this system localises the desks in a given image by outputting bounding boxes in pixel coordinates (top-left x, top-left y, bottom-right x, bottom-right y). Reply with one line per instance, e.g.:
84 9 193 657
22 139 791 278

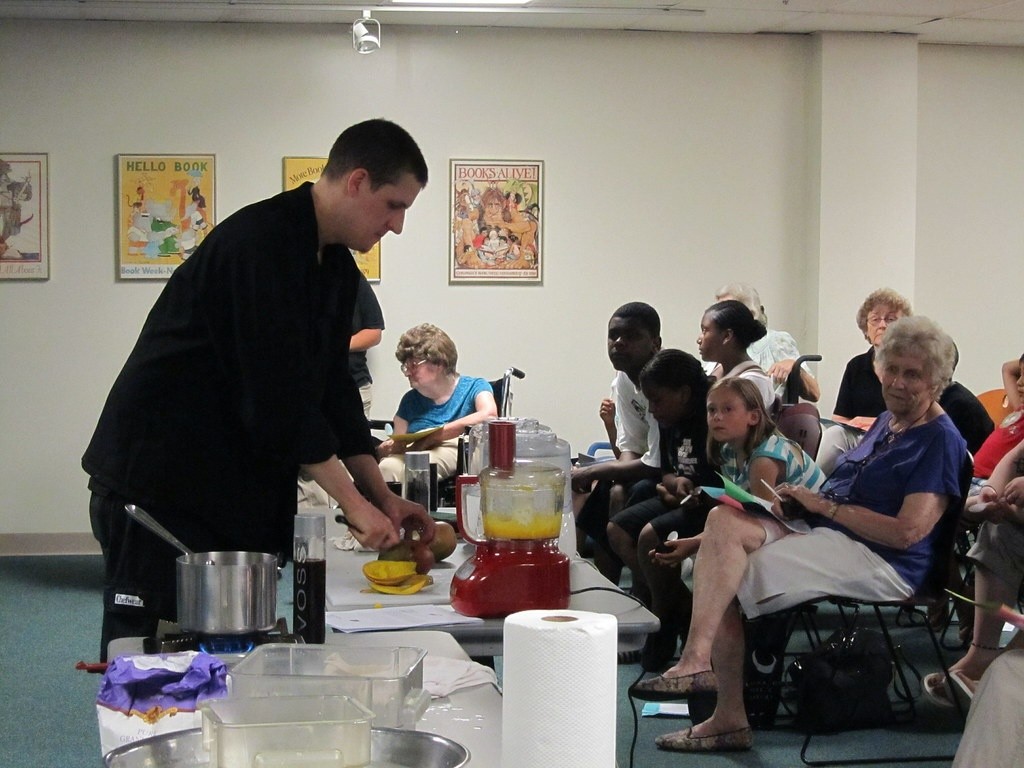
102 629 504 768
295 503 662 656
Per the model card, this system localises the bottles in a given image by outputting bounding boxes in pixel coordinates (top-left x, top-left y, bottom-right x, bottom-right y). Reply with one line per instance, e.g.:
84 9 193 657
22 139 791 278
468 418 577 561
405 451 430 515
293 512 326 644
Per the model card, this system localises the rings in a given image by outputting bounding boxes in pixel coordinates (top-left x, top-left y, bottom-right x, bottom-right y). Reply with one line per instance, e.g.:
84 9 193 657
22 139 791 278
785 482 789 489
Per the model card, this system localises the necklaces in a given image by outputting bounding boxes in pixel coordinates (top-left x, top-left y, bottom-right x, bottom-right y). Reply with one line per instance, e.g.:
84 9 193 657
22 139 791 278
887 401 933 444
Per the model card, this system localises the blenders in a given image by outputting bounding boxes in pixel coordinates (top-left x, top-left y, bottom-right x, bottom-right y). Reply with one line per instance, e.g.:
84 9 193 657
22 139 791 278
449 460 570 616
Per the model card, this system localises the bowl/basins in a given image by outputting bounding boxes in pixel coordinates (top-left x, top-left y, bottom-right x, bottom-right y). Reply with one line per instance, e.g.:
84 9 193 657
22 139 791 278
104 727 471 768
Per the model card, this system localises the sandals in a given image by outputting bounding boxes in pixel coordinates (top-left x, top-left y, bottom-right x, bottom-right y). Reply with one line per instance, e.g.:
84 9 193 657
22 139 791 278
944 670 975 714
921 673 954 708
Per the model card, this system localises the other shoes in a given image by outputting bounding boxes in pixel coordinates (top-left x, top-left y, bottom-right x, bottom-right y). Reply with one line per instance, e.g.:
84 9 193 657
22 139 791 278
953 585 976 640
640 617 679 673
925 586 952 631
655 727 752 754
630 669 720 701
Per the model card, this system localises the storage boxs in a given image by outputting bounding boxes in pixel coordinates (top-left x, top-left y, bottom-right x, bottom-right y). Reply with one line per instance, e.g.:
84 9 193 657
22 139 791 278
192 691 377 768
226 641 432 731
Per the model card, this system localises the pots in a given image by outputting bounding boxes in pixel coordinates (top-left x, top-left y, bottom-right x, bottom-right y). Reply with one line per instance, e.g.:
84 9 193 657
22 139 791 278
176 552 278 634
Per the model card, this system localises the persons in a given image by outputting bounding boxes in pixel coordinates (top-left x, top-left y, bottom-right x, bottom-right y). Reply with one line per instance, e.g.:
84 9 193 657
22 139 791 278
80 117 436 663
595 348 723 665
296 268 385 519
950 648 1024 768
922 351 1024 713
599 286 1024 565
332 322 498 551
652 377 833 594
570 303 662 589
628 318 969 750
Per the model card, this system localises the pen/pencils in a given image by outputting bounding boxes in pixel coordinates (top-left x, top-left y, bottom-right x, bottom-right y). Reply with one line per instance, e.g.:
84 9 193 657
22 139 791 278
680 494 693 505
759 478 784 503
610 392 613 399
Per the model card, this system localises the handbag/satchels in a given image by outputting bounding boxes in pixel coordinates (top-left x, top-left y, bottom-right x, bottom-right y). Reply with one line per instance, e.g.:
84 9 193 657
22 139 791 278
787 624 900 734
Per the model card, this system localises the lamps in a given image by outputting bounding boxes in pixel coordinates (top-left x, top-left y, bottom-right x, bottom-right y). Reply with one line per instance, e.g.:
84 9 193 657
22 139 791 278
351 9 382 56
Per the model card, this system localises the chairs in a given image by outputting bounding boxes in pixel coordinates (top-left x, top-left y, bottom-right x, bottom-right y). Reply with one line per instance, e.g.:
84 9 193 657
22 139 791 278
756 450 967 762
778 403 821 466
972 389 1015 427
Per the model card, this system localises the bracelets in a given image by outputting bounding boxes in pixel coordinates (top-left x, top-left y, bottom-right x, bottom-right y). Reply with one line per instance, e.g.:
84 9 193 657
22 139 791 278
831 503 840 520
982 485 998 501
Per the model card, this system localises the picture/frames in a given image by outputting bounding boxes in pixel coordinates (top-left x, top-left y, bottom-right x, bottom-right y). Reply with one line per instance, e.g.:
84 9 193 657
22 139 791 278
448 158 544 290
280 153 383 285
0 150 51 286
115 151 218 283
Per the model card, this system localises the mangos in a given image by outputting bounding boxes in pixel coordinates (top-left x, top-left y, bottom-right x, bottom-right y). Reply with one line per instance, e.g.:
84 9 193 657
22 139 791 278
378 521 457 575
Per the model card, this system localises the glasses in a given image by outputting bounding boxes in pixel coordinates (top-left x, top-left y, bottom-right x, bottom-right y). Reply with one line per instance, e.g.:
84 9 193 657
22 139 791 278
865 314 899 328
401 359 428 373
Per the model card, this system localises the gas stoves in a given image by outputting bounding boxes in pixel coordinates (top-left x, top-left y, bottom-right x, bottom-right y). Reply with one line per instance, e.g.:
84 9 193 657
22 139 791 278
143 617 297 655
